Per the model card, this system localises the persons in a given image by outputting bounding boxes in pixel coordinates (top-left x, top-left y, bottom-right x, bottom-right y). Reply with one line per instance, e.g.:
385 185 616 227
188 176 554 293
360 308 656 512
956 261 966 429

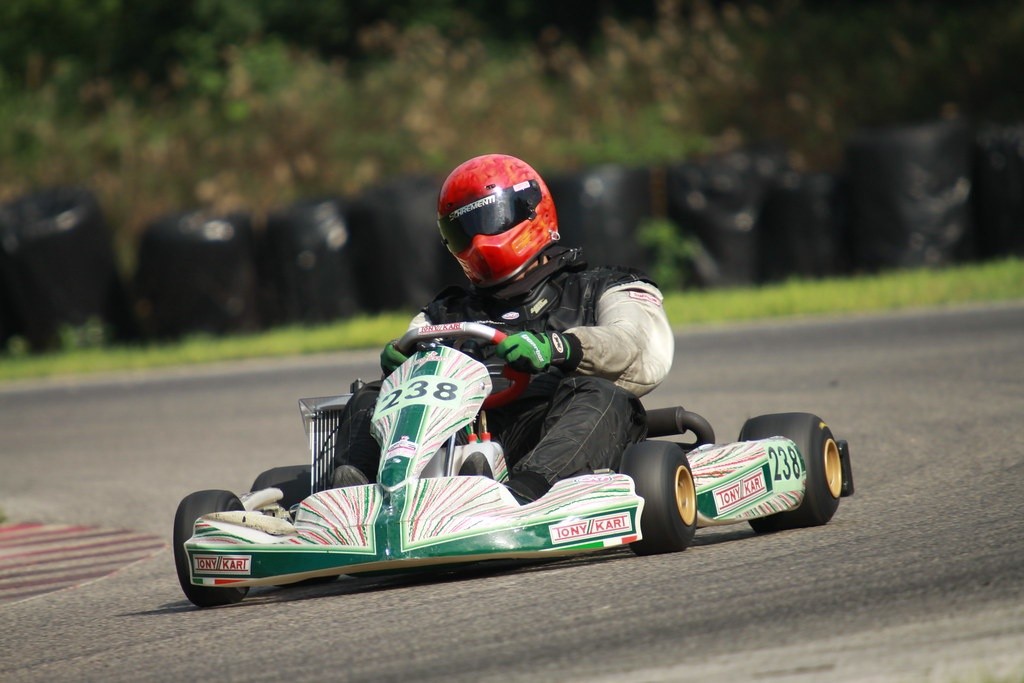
324 151 677 502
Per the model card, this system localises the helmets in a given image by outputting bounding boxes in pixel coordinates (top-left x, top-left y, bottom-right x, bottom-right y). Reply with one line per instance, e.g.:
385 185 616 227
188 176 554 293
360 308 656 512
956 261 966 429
435 152 559 292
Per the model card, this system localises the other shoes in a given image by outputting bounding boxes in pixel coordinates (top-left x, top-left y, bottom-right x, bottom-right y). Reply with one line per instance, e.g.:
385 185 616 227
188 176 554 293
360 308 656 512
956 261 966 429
505 480 537 505
331 465 368 488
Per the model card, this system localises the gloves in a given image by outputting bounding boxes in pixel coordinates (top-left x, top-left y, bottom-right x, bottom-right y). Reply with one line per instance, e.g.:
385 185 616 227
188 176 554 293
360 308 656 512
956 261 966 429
496 329 574 375
381 340 408 376
